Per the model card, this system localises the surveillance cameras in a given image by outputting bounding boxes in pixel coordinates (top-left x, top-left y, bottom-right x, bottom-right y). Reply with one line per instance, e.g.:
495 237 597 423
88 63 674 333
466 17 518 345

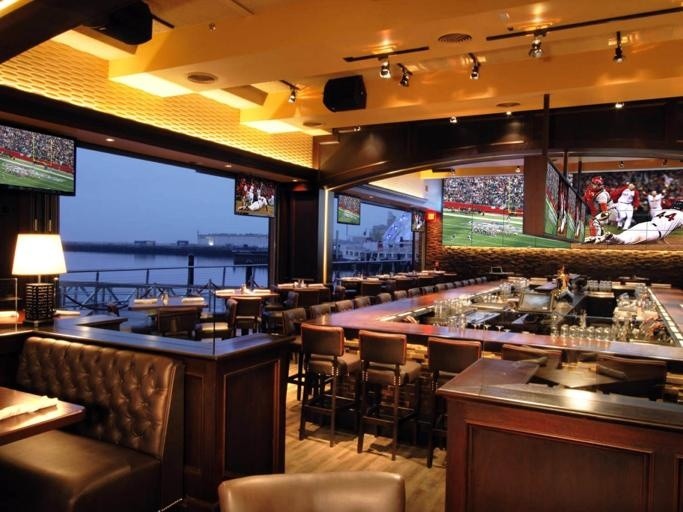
380 60 393 81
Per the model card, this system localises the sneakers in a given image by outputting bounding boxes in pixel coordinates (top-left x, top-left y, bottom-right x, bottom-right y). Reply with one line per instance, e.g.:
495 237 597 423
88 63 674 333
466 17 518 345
585 231 613 243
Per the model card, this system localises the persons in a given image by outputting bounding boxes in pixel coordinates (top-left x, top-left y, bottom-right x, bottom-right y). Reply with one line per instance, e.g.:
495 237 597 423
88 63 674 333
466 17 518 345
235 175 278 218
0 125 75 186
412 168 683 248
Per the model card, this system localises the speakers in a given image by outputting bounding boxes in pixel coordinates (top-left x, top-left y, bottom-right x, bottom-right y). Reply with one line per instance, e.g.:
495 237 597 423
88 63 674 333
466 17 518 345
323 75 368 113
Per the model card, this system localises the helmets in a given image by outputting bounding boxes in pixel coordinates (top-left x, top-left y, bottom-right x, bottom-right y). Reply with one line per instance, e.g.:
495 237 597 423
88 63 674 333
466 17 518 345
671 200 683 210
591 177 603 191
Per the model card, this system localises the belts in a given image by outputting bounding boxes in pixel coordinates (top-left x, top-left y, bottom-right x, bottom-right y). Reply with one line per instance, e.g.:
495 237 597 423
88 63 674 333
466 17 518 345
607 199 611 204
620 203 631 204
653 223 661 238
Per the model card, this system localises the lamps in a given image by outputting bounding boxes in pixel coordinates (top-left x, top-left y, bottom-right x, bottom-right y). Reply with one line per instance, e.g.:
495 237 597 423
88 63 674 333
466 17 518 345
10 233 69 330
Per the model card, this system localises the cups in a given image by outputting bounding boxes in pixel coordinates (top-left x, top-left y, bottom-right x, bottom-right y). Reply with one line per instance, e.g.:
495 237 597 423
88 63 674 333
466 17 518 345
445 315 467 335
585 280 611 292
432 296 470 318
498 283 511 297
511 276 529 290
474 292 503 304
548 324 614 342
549 338 611 349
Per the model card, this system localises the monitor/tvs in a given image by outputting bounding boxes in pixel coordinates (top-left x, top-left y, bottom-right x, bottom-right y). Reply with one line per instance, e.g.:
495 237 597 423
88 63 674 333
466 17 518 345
0 121 78 197
519 292 555 315
560 176 569 240
568 186 576 242
575 194 582 241
234 172 278 219
581 201 587 241
337 193 361 225
542 158 560 237
410 209 426 232
